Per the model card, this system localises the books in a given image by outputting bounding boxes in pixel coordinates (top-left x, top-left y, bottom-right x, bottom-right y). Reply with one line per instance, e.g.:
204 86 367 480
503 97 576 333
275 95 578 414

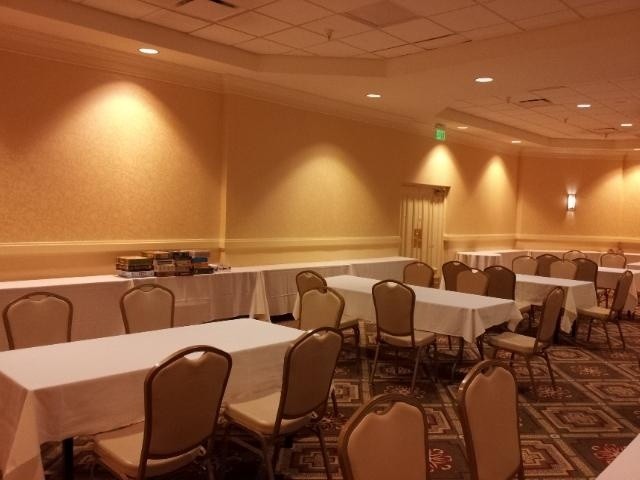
115 248 214 278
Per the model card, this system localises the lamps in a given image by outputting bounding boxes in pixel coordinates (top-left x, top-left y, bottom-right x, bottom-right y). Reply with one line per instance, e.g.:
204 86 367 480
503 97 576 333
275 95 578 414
566 194 576 211
